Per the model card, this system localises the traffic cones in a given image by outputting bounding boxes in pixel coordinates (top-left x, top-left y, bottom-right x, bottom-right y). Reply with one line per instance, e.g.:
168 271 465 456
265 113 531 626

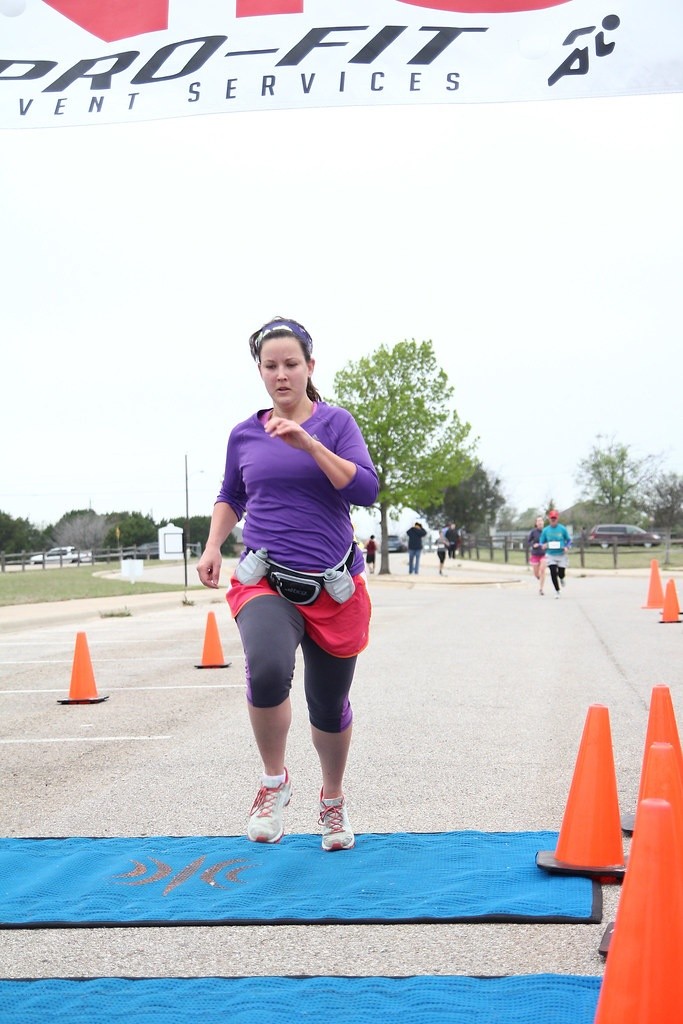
657 579 683 624
193 612 232 668
535 703 630 878
56 631 109 703
593 683 683 1024
642 559 663 608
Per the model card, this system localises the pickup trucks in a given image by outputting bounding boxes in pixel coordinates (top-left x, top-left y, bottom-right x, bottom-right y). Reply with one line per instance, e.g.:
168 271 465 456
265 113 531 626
30 546 92 564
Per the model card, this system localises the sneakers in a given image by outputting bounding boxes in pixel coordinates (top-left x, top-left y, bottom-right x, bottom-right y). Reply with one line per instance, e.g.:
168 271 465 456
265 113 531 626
248 768 292 844
318 786 354 851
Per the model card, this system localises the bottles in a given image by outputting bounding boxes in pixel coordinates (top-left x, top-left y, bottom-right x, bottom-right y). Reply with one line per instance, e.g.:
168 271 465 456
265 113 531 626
325 568 342 581
255 547 268 563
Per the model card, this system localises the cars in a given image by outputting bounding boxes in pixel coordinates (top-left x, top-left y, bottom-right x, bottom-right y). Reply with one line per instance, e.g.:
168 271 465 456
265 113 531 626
123 542 159 560
587 525 661 549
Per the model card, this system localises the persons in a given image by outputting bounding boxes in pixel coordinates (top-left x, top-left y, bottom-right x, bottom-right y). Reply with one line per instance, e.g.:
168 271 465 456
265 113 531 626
195 315 380 851
539 510 571 599
436 530 449 574
407 522 426 573
527 516 546 595
366 535 376 573
446 522 459 558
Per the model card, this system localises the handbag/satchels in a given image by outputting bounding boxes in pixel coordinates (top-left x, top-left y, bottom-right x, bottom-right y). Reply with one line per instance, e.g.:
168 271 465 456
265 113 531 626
245 546 355 604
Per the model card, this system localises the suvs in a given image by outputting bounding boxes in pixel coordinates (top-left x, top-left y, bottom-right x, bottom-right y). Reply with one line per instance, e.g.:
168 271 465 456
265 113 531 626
380 534 406 552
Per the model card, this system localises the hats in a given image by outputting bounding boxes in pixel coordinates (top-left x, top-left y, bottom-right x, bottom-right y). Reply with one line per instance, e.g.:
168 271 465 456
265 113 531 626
549 510 559 519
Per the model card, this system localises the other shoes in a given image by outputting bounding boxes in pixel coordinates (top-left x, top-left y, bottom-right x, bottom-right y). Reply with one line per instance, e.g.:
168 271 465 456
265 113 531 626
560 577 567 586
555 590 563 600
539 589 545 596
537 576 540 579
